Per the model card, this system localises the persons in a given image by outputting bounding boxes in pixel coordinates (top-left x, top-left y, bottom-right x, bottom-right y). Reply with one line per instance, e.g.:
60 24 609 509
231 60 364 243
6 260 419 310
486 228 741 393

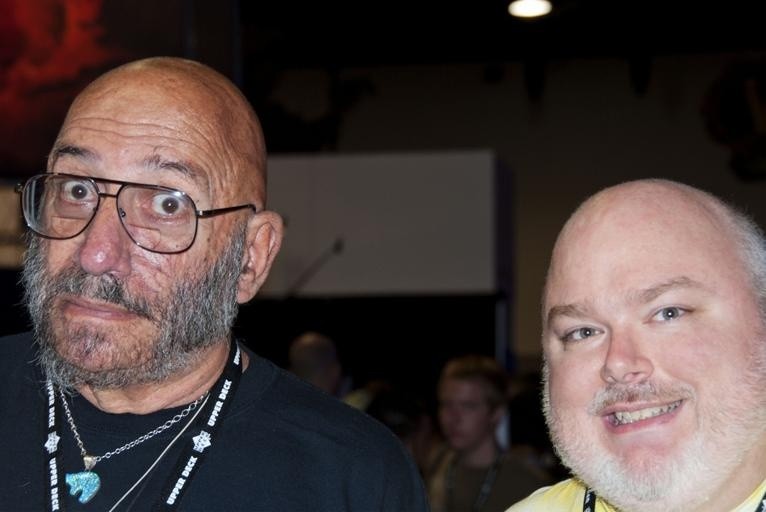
422 358 552 512
501 177 765 512
1 57 429 511
286 331 341 400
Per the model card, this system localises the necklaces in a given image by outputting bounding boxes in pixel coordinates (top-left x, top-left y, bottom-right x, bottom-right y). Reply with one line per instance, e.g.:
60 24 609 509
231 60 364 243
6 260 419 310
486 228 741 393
53 376 208 504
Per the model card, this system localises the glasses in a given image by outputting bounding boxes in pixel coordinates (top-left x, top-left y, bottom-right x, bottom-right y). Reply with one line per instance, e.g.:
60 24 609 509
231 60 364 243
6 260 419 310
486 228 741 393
11 171 257 256
